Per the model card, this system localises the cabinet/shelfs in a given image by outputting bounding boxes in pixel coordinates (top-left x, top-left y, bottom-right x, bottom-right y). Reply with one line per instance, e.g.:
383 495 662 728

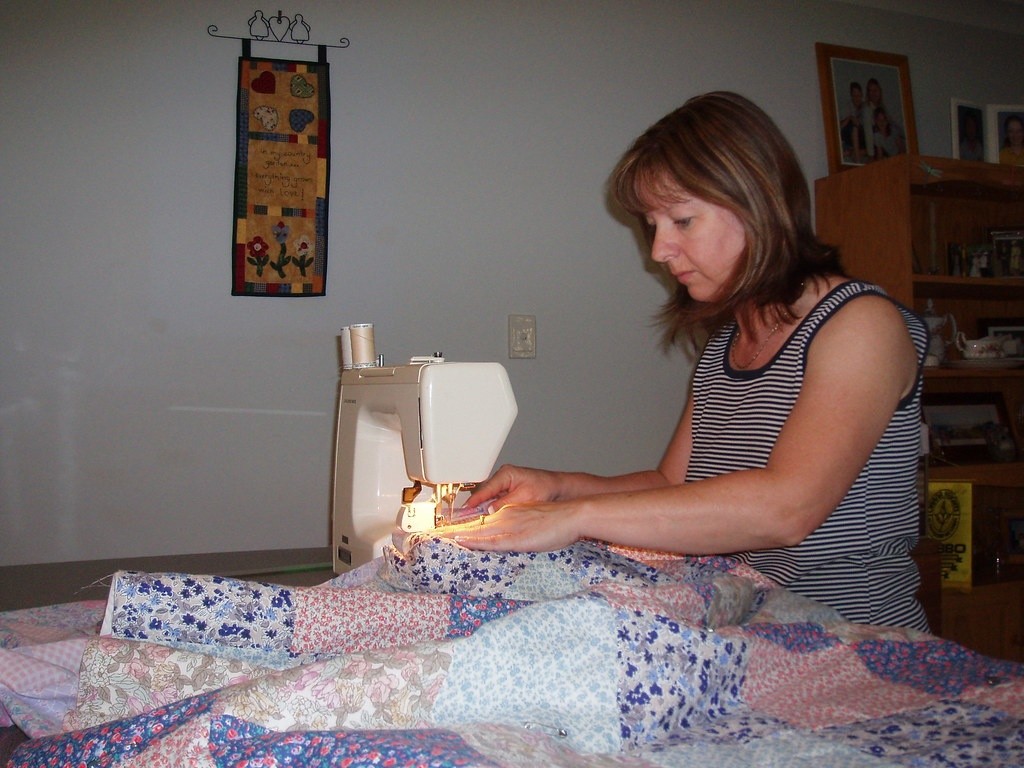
814 155 1024 659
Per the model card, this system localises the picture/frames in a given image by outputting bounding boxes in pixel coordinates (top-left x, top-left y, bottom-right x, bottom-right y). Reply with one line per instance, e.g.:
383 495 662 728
988 104 1024 165
815 42 919 174
985 225 1024 279
950 97 988 161
977 317 1024 357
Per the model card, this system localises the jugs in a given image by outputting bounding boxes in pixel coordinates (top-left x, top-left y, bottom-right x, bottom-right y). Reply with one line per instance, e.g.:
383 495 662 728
921 299 957 361
956 331 1011 359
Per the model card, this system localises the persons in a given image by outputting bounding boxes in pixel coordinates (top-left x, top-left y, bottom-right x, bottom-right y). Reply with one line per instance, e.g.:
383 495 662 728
454 91 931 636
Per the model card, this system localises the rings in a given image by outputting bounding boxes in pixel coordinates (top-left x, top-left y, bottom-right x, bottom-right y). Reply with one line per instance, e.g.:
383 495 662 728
480 514 485 524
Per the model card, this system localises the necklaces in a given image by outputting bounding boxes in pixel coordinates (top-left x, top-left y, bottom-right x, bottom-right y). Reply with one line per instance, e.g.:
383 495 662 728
732 310 787 369
